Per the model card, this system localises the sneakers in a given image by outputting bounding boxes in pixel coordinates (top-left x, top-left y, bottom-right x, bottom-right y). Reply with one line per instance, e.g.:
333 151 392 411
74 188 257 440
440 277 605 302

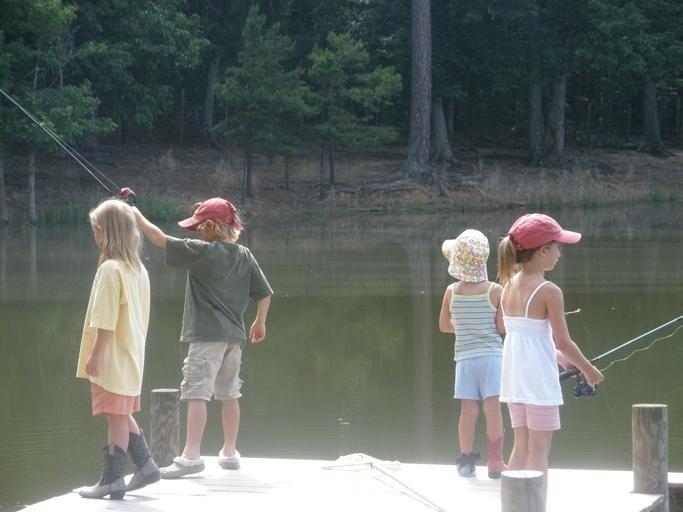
457 452 482 478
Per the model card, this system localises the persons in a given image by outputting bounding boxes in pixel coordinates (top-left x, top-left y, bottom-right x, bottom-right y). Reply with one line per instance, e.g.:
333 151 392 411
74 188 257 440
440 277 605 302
119 181 273 481
436 226 506 478
495 212 607 487
71 194 160 503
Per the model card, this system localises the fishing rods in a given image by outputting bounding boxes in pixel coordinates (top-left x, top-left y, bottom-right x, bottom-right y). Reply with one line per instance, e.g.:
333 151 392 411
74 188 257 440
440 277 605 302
1 87 118 198
560 316 683 398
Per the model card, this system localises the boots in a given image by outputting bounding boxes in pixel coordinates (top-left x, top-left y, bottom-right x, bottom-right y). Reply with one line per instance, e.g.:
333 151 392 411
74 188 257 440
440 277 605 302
77 441 130 503
486 429 510 481
124 428 161 493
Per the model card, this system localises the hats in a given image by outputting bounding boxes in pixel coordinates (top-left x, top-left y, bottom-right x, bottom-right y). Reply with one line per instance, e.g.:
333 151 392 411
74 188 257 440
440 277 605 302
440 227 491 285
177 196 244 231
507 212 583 252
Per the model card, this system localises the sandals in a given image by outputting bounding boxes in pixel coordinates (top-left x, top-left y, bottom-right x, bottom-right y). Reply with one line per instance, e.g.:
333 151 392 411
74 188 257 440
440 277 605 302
216 446 242 472
157 456 205 480
